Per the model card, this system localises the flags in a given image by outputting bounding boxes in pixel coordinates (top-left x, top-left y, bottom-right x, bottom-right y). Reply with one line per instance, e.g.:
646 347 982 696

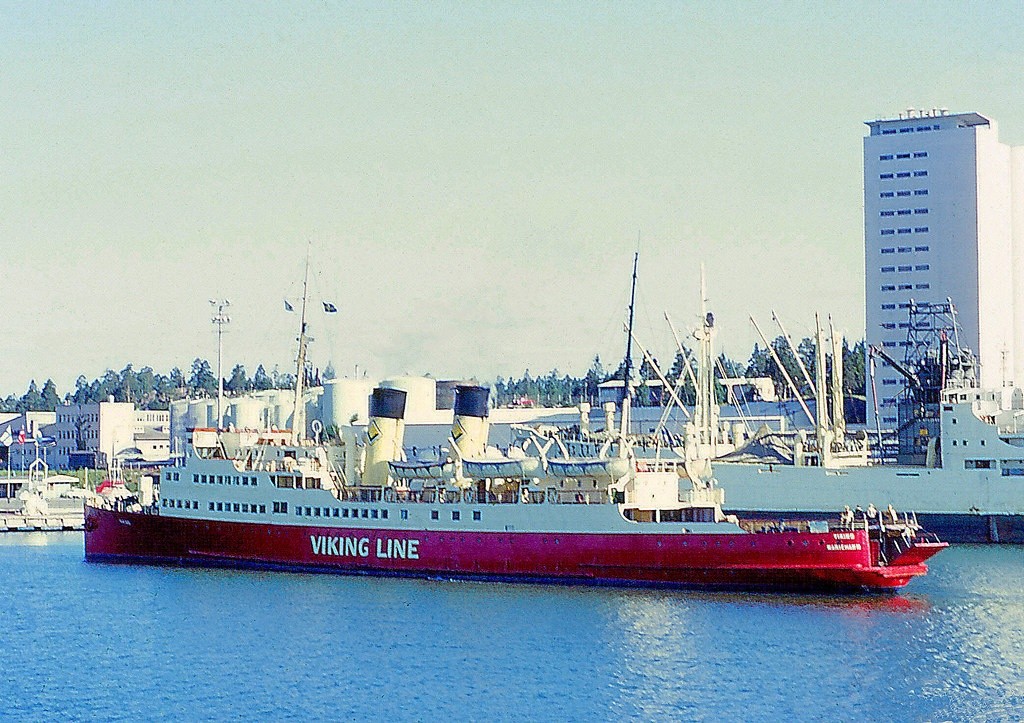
35 430 43 444
285 301 293 311
18 425 25 444
0 425 13 446
324 302 336 313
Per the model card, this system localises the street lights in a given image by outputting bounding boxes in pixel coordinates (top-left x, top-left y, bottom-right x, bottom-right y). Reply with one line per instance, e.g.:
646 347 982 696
210 299 232 428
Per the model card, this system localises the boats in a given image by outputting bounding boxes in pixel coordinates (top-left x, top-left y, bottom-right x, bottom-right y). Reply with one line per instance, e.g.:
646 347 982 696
510 296 1023 546
84 231 949 593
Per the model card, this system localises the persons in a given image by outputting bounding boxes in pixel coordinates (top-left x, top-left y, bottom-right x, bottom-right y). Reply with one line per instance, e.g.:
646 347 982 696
577 490 587 505
542 425 581 441
520 488 529 504
804 442 817 465
489 491 497 503
439 488 445 504
101 495 143 514
841 504 897 527
